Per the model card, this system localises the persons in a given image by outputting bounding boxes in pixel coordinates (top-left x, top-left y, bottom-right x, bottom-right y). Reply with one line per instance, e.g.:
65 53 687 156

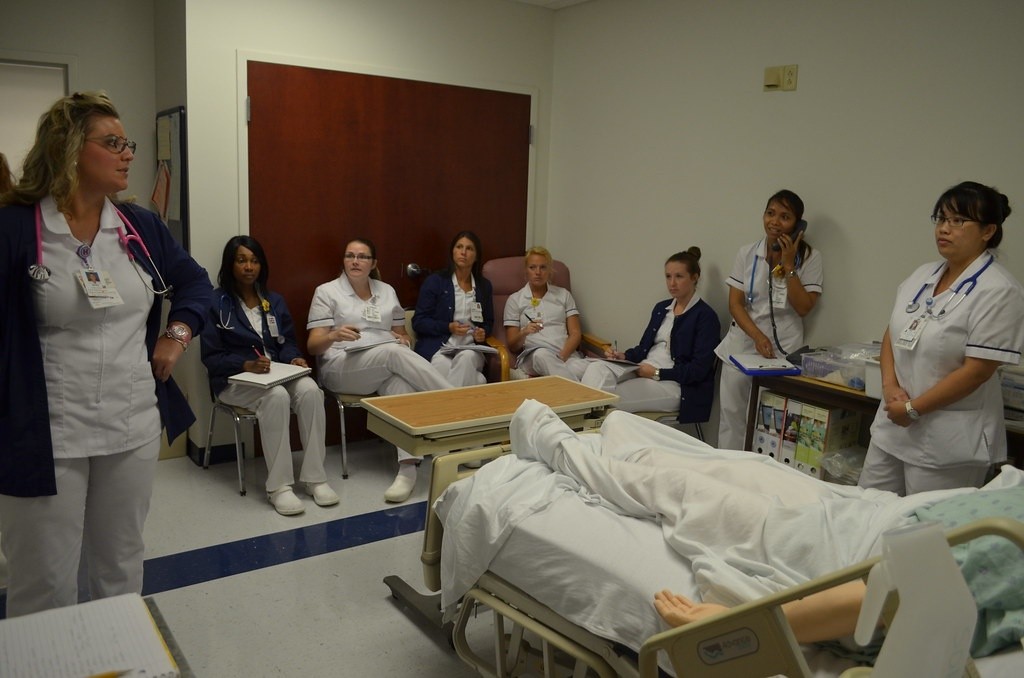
714 189 824 451
503 246 590 384
0 91 214 620
857 181 1024 497
411 231 493 468
200 235 339 516
307 237 457 502
509 399 1024 660
579 246 721 425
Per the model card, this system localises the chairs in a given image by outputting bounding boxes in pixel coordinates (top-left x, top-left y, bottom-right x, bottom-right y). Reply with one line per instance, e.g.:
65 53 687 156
483 257 617 382
204 304 422 497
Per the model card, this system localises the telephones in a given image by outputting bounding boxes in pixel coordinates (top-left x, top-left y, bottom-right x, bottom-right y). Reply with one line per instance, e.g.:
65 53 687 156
774 218 807 250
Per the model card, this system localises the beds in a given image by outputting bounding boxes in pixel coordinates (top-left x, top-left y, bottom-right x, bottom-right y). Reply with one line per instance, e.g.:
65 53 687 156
382 427 1024 678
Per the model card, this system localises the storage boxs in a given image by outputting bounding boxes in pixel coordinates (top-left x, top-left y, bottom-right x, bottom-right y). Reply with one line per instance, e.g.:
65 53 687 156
753 389 863 480
799 342 883 400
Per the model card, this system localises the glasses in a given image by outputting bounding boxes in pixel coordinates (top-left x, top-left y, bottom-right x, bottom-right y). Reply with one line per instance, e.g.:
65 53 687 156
930 213 980 228
85 134 136 155
345 253 373 262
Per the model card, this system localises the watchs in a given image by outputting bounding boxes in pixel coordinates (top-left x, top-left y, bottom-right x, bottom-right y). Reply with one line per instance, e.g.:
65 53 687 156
652 368 660 381
905 400 921 421
165 324 192 353
783 269 797 278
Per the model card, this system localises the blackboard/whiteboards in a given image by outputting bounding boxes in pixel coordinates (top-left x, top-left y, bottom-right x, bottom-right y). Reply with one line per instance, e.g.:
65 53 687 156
0 59 71 182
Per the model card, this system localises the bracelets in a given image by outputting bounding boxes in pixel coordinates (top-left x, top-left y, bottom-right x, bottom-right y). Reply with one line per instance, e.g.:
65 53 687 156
405 340 411 349
556 354 567 362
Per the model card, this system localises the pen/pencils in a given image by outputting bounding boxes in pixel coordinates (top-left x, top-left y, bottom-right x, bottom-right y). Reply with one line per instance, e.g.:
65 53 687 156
251 343 264 360
523 313 535 323
614 341 617 357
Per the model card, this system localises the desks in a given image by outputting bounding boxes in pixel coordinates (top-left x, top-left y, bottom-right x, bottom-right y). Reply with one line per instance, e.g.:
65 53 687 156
359 372 620 460
744 372 1024 483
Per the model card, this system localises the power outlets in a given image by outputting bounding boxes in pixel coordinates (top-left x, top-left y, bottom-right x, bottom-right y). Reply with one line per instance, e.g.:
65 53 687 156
783 64 797 91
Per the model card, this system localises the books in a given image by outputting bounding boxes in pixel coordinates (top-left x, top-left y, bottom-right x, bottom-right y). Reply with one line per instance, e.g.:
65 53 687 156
228 360 312 390
585 356 642 379
343 338 401 355
439 341 499 355
515 345 566 366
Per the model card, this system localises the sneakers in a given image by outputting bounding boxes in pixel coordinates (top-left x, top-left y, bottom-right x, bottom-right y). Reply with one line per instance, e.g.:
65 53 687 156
384 462 415 502
267 484 305 515
304 482 338 505
